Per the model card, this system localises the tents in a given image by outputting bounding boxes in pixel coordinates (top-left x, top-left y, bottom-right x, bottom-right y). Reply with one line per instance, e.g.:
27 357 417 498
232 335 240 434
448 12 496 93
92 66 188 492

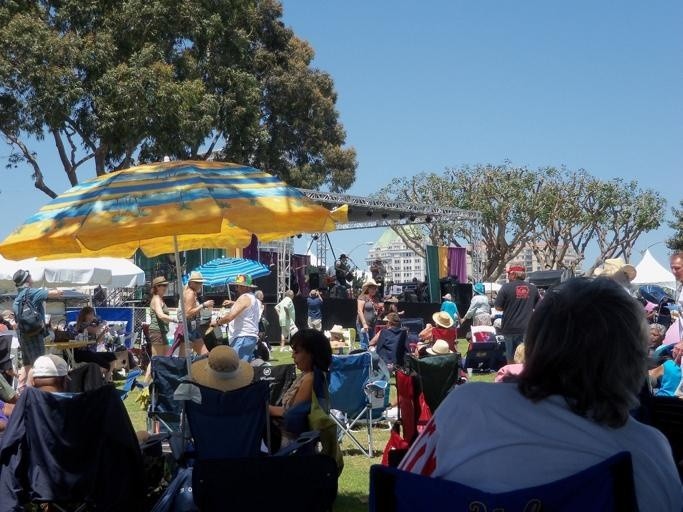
530 271 571 289
630 249 678 296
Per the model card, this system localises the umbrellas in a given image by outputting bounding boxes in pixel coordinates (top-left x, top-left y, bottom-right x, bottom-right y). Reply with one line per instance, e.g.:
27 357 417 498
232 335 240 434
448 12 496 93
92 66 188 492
0 157 347 377
1 256 145 288
182 257 272 301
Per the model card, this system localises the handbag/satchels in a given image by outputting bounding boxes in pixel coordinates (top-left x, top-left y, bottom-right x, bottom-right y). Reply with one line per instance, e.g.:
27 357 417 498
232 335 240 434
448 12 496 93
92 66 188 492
308 386 345 478
53 318 70 343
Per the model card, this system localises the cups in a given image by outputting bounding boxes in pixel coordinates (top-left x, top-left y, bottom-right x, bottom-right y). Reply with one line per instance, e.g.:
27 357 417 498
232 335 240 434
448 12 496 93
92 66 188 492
146 417 154 436
154 421 160 435
207 300 214 316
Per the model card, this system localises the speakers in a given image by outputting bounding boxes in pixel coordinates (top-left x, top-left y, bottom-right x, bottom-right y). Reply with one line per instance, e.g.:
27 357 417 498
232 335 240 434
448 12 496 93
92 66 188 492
441 283 473 331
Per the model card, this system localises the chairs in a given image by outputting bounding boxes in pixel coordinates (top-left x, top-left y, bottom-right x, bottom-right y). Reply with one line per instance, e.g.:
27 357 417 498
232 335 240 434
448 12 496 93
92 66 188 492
252 365 296 427
390 354 465 447
401 319 423 342
327 353 391 458
370 451 637 512
462 342 497 373
153 378 338 512
21 387 171 512
147 356 203 436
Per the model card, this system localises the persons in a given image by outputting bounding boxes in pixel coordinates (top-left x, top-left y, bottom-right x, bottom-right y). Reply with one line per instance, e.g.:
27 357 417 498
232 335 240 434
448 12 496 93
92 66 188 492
335 254 349 286
670 251 683 316
649 344 683 396
12 270 61 397
398 278 683 512
370 257 386 297
29 354 150 449
495 341 525 383
466 332 472 352
356 279 384 351
460 283 492 326
175 271 213 356
426 340 467 385
415 311 458 359
268 329 333 449
648 323 665 346
274 290 295 352
375 313 413 366
254 291 266 341
306 289 323 332
210 275 258 364
439 293 461 326
143 276 177 386
0 401 13 431
179 345 282 453
74 306 117 383
0 373 19 404
381 294 405 314
0 313 13 332
2 309 16 325
592 258 636 295
494 261 542 365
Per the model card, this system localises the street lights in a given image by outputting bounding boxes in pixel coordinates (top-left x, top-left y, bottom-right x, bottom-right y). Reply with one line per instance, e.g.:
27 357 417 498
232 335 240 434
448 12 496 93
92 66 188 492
326 242 373 257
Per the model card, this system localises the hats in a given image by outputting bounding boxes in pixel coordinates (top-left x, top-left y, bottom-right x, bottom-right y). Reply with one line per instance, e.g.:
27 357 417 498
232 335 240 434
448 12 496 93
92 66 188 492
12 269 30 287
425 339 454 356
229 273 258 288
432 310 454 328
187 270 207 283
472 282 486 295
31 353 69 377
442 293 452 300
308 289 318 298
189 344 255 392
361 278 382 288
387 296 399 303
152 276 169 285
337 254 349 259
506 266 525 273
593 258 637 281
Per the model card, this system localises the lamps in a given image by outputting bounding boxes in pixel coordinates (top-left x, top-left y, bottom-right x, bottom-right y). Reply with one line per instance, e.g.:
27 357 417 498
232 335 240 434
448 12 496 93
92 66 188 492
409 212 416 222
425 216 432 223
311 234 318 240
